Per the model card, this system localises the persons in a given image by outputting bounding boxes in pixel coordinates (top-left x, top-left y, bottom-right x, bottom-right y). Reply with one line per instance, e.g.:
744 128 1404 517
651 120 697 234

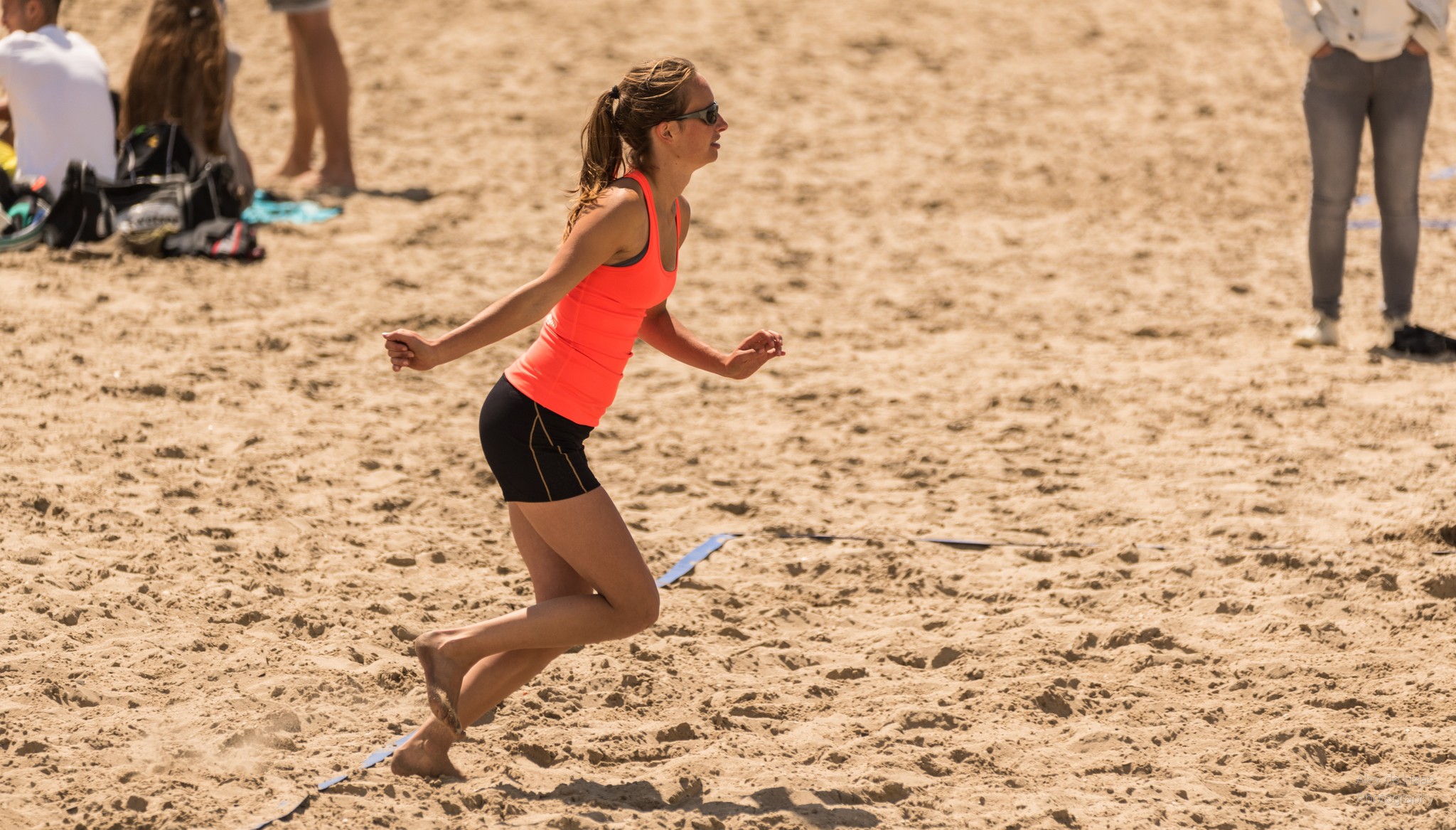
1274 0 1451 347
0 0 119 227
112 0 255 226
384 57 785 782
261 0 360 199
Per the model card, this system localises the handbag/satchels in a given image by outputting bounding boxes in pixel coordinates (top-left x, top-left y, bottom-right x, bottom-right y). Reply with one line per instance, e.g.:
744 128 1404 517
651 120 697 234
46 157 241 250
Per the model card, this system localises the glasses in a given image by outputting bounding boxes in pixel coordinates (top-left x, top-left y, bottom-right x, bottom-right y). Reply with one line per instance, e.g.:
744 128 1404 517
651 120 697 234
642 101 719 131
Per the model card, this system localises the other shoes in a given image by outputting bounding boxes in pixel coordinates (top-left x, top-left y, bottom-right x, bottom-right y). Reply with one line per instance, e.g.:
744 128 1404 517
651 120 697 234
1293 311 1341 348
1378 315 1410 349
